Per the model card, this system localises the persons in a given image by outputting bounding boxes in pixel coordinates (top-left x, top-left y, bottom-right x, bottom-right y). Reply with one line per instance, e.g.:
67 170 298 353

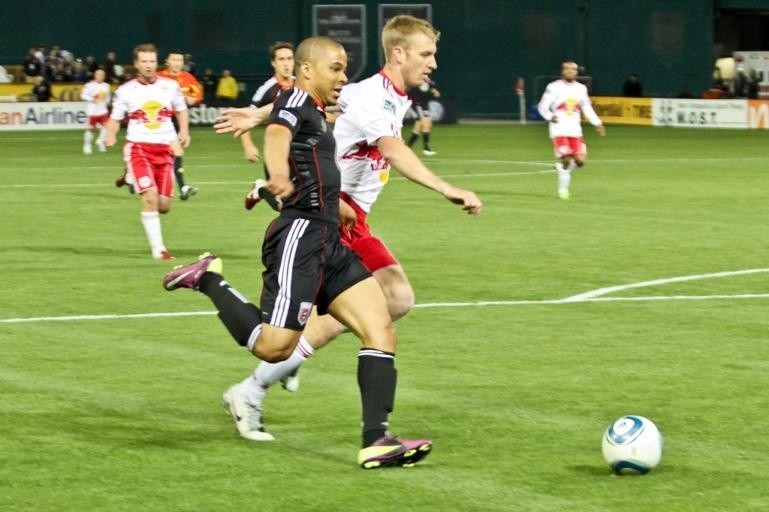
113 51 204 200
537 60 606 200
161 36 434 470
713 54 764 99
100 44 190 262
212 14 483 443
622 72 643 97
23 45 239 155
238 41 296 212
577 65 590 94
405 74 440 156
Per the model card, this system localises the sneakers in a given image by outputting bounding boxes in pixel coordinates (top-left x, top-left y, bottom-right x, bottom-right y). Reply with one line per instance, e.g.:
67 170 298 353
94 138 107 153
358 432 433 468
282 371 301 394
245 178 268 210
222 382 275 443
163 252 224 292
422 149 436 155
83 141 92 155
558 187 570 200
179 185 198 200
116 167 127 188
151 250 177 263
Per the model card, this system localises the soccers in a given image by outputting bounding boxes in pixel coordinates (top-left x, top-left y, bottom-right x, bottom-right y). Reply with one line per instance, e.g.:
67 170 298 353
602 416 662 476
